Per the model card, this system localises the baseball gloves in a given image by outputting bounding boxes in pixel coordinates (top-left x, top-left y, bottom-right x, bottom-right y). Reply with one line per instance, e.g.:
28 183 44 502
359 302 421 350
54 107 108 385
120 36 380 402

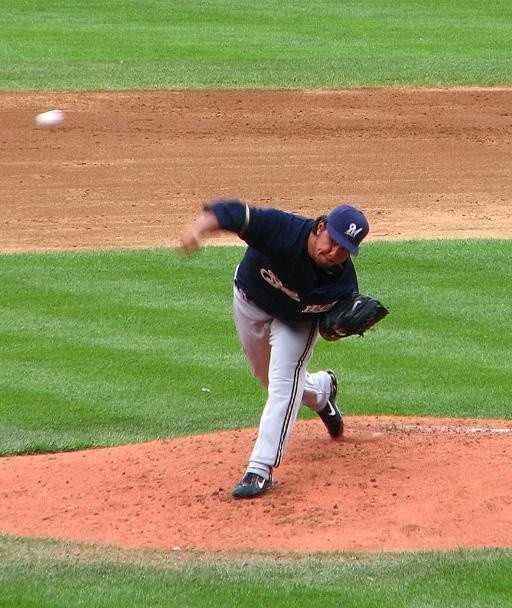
323 291 388 337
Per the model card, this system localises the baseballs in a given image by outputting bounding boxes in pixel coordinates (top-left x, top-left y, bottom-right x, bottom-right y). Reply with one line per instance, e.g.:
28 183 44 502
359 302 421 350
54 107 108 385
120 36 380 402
35 110 64 127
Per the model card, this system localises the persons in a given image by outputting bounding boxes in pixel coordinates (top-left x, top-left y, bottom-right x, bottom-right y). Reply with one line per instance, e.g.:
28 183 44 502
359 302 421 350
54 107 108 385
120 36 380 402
176 199 388 499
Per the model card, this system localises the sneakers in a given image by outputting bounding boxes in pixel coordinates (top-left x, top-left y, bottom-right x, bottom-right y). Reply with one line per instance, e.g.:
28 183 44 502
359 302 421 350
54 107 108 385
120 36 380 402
316 370 344 440
231 470 272 499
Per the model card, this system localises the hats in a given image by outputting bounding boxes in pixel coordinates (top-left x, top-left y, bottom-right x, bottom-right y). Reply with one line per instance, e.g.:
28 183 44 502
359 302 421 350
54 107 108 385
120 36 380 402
324 202 370 256
